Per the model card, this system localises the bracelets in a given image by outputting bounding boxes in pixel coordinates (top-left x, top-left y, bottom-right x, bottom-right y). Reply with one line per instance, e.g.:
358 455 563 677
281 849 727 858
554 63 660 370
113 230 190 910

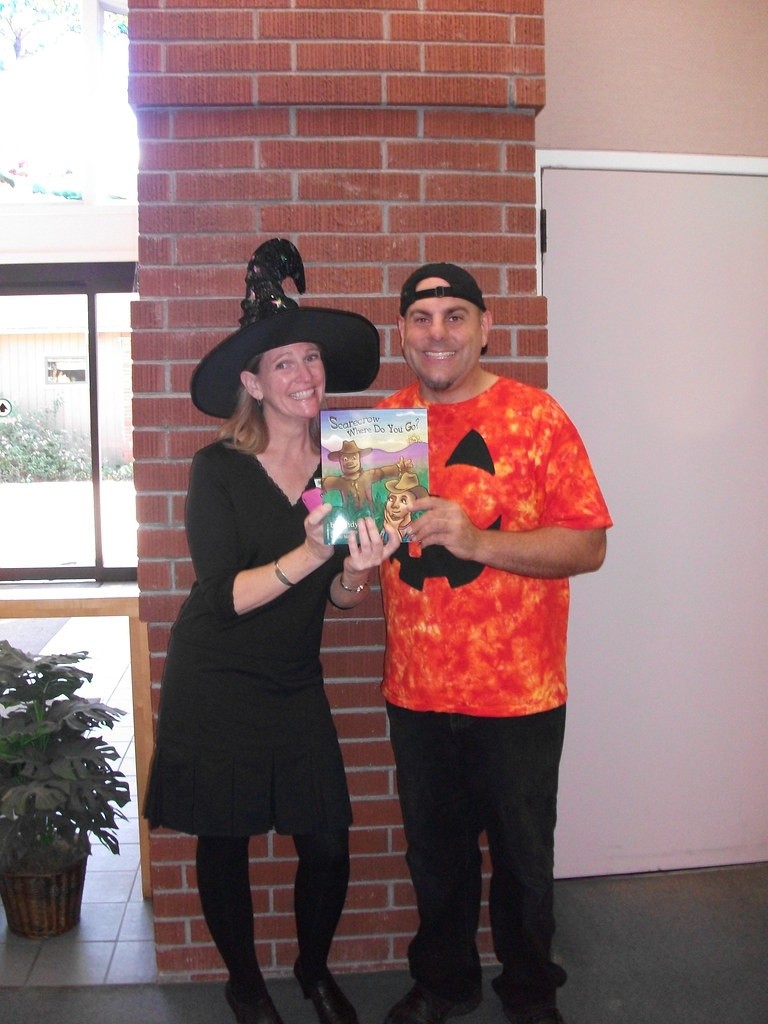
274 560 297 587
339 575 369 594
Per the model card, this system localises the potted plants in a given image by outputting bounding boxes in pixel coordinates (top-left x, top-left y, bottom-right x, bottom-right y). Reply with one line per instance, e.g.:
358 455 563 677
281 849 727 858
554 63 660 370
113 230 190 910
0 639 131 939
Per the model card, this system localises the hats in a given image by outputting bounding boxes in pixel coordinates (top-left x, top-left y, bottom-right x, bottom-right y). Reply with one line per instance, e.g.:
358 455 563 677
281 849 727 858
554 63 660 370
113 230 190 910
190 237 381 418
399 262 487 356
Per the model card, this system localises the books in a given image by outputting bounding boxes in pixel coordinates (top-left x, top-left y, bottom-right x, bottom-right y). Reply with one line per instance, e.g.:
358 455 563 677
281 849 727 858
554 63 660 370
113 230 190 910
320 409 429 544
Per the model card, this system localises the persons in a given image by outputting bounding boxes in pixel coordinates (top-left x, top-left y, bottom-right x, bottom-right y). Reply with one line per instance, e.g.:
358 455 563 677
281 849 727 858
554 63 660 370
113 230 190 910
372 261 612 1024
141 239 402 1024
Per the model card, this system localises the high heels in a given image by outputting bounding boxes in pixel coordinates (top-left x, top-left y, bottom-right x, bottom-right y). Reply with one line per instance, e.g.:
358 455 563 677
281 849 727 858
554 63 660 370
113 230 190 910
225 979 283 1024
294 956 359 1024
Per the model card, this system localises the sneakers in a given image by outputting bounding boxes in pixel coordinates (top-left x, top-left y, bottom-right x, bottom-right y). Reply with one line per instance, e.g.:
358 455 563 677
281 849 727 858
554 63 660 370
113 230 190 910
502 992 565 1024
383 980 482 1024
